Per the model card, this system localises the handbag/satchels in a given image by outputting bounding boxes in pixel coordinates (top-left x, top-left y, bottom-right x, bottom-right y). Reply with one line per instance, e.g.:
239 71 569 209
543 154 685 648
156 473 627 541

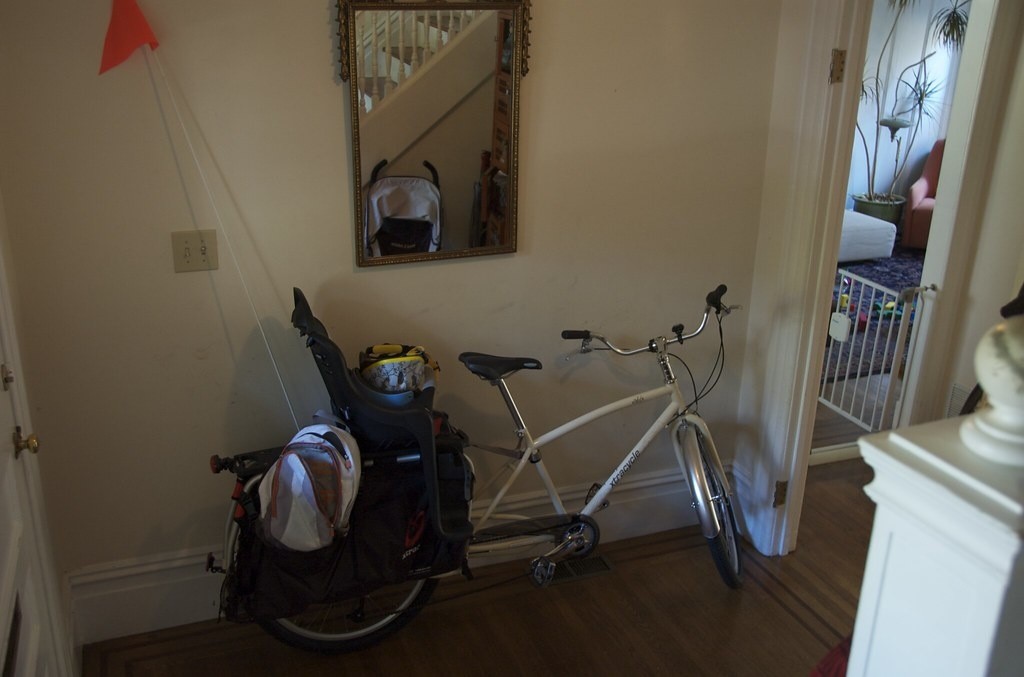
259 407 359 555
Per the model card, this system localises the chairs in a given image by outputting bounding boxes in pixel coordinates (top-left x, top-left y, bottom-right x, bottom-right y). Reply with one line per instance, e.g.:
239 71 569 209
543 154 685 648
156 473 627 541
291 287 474 540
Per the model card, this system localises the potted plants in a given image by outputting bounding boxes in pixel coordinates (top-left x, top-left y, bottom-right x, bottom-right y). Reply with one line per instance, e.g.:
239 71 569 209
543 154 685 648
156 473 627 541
857 0 972 226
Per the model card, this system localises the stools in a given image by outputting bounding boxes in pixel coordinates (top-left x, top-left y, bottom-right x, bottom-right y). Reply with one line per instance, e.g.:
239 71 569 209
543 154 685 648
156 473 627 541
838 209 897 261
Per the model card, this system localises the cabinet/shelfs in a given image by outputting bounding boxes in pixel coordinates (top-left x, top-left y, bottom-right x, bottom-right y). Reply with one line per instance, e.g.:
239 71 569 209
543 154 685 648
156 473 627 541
484 10 514 246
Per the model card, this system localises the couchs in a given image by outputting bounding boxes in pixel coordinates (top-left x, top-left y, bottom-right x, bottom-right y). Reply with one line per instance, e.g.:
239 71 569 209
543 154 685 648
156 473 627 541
901 140 945 257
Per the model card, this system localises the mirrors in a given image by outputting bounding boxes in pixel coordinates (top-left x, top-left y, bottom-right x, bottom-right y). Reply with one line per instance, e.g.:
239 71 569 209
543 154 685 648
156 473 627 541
335 0 532 269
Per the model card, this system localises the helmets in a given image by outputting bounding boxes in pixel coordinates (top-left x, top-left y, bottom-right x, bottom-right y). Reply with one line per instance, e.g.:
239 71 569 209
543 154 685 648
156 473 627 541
353 345 439 410
364 356 425 392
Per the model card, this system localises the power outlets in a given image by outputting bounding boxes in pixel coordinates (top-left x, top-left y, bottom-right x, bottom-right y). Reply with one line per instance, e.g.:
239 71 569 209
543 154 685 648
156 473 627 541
171 230 219 272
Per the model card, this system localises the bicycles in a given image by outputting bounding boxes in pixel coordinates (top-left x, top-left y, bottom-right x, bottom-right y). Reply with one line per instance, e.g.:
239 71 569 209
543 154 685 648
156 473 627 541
212 283 745 654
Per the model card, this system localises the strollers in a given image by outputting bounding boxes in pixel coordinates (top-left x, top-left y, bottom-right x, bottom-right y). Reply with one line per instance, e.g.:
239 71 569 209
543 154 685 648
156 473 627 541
365 159 443 256
